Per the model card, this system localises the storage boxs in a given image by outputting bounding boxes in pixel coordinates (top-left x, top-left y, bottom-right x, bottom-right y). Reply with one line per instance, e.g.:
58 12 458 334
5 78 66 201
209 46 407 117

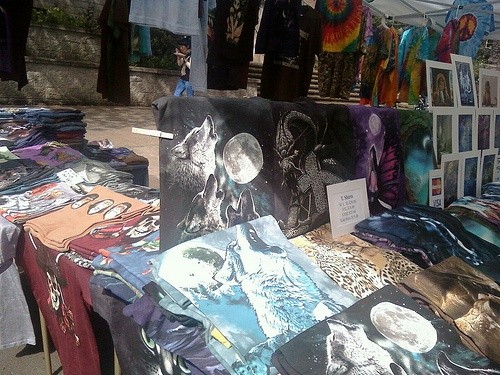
89 147 149 187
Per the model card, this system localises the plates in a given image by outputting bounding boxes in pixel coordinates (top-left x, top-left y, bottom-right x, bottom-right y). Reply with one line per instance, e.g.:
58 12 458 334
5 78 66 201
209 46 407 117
173 53 186 57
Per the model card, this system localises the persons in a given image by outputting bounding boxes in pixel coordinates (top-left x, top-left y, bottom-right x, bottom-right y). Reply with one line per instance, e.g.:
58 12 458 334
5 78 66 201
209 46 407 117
174 38 194 97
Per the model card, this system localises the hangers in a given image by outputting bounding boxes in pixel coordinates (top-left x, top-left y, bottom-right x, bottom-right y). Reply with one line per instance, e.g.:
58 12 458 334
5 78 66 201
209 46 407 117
455 4 461 21
377 17 390 29
